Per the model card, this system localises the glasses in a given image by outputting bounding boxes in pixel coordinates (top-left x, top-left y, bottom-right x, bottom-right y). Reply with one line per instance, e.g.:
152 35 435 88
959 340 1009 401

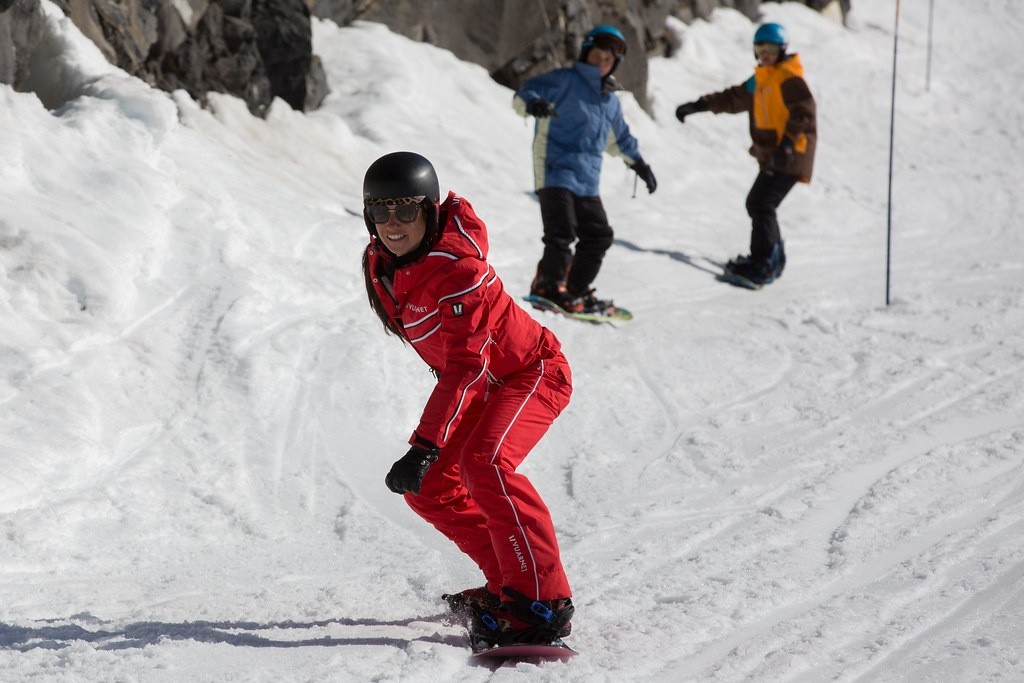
366 201 423 224
753 44 780 56
591 32 626 57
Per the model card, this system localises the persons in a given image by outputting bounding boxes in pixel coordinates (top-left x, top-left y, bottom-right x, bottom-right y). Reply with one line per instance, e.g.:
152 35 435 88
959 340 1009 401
503 25 658 327
676 23 819 292
360 152 574 661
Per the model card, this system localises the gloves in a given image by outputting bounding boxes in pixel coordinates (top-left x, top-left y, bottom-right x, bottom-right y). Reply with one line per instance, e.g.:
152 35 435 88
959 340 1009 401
630 158 657 194
774 145 794 170
385 444 439 494
525 97 549 117
676 98 709 123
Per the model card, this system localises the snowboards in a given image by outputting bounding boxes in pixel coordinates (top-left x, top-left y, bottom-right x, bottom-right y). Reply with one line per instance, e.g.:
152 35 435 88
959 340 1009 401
443 591 580 659
717 270 764 291
521 293 634 324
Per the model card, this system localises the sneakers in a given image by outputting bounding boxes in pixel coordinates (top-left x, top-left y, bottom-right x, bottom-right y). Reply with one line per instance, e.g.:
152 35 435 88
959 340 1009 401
471 593 575 645
446 586 502 618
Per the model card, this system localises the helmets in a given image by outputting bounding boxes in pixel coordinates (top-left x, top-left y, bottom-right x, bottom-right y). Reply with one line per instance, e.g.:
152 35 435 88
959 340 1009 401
584 24 627 62
363 151 439 223
753 23 789 45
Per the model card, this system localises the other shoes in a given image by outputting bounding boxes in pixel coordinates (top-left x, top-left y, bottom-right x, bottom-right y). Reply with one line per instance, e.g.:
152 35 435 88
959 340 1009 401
573 288 613 312
726 244 787 284
530 277 584 312
